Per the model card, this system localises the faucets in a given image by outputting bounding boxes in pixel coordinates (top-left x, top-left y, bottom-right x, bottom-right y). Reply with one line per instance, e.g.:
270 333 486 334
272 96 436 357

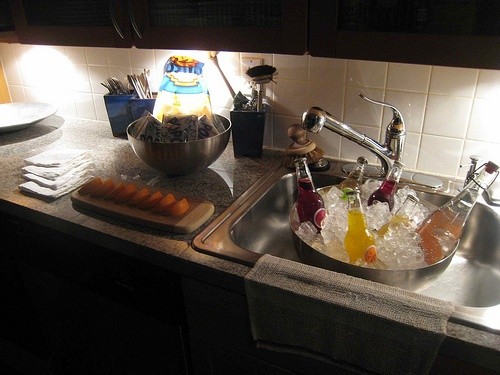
300 92 407 179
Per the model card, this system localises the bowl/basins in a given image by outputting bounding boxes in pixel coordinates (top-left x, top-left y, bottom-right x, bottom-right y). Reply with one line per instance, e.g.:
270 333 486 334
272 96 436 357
288 184 460 290
127 115 231 180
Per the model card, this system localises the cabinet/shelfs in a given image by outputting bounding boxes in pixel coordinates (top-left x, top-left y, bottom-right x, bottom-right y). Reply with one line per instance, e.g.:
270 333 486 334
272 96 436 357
0 210 193 375
0 0 500 70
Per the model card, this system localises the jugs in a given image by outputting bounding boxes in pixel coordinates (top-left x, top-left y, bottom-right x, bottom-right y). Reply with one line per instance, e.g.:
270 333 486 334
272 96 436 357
154 54 212 121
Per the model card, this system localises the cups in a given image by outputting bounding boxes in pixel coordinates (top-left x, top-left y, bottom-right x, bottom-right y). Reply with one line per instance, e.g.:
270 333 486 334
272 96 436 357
102 88 134 141
230 107 268 158
131 91 159 120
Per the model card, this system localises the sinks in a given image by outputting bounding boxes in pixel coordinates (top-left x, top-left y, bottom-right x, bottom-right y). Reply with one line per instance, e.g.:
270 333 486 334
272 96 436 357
190 156 500 335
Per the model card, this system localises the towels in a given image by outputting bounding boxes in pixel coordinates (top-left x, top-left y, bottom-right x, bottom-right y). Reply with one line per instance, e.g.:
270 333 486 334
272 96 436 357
18 174 95 199
21 160 94 190
24 149 93 168
22 157 92 180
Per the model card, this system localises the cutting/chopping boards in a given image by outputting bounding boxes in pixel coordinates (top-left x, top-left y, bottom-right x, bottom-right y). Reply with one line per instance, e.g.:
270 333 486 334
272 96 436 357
70 181 215 233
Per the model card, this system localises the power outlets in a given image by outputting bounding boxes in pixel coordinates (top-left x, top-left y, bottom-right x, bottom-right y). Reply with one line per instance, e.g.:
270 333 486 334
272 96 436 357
241 57 263 97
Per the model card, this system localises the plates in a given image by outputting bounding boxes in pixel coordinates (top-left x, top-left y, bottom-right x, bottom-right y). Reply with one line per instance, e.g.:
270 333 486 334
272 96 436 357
0 103 56 134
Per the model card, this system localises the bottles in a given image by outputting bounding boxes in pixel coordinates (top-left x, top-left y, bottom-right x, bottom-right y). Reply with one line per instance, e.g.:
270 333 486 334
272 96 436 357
336 156 370 203
343 188 377 264
293 156 328 233
418 161 500 265
378 194 419 238
369 159 403 212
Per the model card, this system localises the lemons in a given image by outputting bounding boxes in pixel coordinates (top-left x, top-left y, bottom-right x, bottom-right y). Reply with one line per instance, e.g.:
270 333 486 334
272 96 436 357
79 177 189 216
